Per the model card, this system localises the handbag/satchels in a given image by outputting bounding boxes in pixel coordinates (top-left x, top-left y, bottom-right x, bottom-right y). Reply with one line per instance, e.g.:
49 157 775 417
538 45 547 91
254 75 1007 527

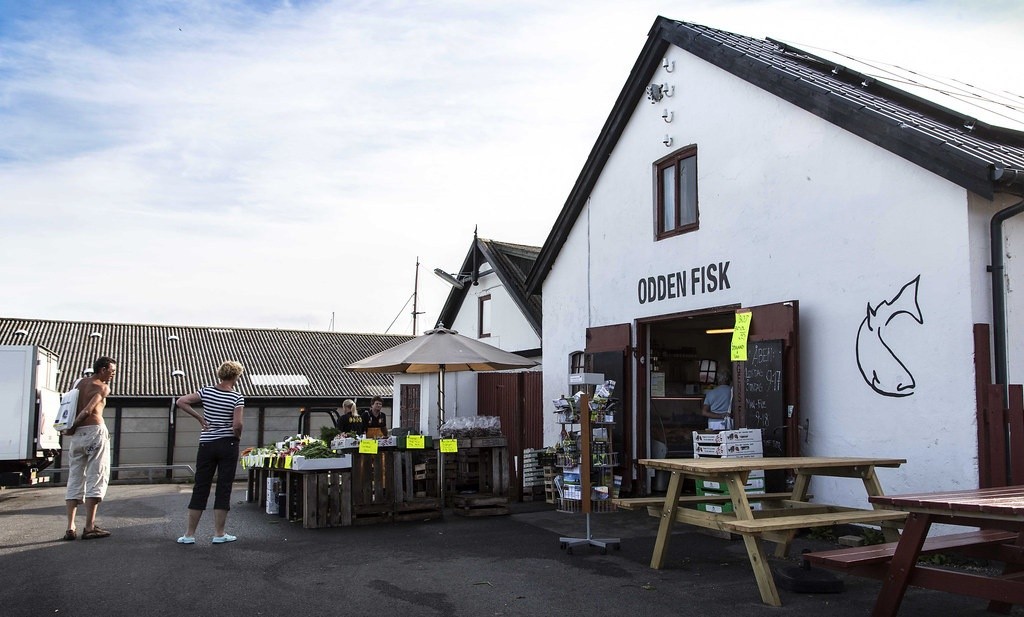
53 377 84 430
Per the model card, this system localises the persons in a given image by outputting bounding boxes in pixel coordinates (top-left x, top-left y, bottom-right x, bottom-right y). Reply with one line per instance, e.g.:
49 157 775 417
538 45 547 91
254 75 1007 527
176 361 245 543
362 396 387 436
63 357 117 541
702 368 734 430
336 399 362 436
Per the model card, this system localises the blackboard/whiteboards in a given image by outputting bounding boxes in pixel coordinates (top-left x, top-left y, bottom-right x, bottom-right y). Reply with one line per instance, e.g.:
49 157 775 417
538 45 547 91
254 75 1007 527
743 339 783 460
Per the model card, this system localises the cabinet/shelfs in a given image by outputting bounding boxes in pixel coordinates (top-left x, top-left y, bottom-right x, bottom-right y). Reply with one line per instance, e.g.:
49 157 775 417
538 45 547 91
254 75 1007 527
543 466 561 504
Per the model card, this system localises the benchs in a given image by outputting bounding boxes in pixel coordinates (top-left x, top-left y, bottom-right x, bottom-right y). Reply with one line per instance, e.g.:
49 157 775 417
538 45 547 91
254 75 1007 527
615 486 1018 571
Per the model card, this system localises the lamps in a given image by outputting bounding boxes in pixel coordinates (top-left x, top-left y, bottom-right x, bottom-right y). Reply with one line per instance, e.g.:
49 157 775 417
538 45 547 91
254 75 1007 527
661 133 673 147
434 267 474 289
663 82 675 97
662 108 673 123
646 84 662 105
662 57 675 73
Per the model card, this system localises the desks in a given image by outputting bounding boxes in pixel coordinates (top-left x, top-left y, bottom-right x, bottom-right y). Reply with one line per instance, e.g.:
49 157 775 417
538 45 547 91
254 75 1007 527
637 454 905 607
867 484 1024 617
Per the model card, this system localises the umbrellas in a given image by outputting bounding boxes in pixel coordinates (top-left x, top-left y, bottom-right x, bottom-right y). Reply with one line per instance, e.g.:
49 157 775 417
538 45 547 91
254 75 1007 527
342 323 541 497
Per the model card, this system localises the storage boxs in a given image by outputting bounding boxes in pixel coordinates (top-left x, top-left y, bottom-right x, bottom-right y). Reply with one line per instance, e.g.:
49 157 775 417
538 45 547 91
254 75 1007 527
265 476 281 515
692 427 769 539
651 371 665 397
276 436 396 470
522 449 582 500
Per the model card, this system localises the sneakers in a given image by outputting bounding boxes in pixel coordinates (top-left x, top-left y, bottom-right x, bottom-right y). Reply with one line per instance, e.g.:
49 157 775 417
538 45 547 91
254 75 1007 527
177 535 195 543
82 525 110 537
64 528 77 540
212 533 237 544
